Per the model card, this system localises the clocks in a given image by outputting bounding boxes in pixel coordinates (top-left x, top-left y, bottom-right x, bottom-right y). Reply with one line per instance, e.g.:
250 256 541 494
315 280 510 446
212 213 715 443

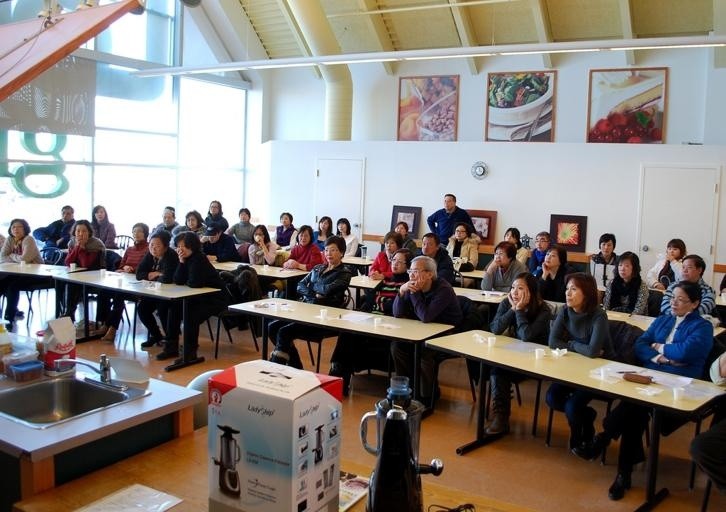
471 161 489 180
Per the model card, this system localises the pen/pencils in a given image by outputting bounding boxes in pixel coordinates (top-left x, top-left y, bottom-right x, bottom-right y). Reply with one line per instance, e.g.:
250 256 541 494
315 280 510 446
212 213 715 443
271 303 288 305
617 372 636 373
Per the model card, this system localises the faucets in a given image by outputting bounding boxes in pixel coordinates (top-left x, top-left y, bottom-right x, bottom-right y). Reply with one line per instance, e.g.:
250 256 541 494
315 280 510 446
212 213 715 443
54 353 111 382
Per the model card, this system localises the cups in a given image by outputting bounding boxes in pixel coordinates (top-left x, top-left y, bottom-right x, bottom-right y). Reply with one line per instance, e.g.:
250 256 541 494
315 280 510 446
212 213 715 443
721 293 726 303
374 317 383 325
535 349 544 359
672 387 684 402
280 304 288 311
21 260 162 292
320 309 327 319
601 368 611 378
264 265 270 273
270 303 277 310
488 336 496 347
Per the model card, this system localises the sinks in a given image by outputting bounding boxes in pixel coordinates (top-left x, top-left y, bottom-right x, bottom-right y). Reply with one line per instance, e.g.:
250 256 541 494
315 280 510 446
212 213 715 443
0 371 152 430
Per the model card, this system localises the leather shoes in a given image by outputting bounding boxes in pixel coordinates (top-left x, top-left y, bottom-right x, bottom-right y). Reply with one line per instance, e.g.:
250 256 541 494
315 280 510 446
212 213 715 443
142 336 179 359
95 324 116 341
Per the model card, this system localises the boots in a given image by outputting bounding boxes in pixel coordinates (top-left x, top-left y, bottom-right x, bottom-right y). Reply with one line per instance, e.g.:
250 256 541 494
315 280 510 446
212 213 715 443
487 375 514 435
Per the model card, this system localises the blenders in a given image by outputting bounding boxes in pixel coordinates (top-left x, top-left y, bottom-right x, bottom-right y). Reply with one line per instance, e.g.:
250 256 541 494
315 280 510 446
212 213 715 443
212 421 242 498
312 425 325 464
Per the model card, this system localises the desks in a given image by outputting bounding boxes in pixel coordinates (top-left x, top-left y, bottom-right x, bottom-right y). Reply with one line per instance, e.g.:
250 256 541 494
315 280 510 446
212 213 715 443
423 329 724 511
227 296 454 420
53 269 223 373
212 254 383 311
1 338 372 510
56 247 126 258
1 262 90 339
450 268 725 338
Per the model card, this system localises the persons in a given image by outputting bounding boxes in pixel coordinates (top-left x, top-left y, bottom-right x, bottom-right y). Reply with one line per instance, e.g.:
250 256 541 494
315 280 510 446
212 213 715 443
368 222 717 314
389 255 463 404
1 199 359 371
483 272 551 433
427 194 476 249
572 280 714 500
544 274 612 446
331 248 416 392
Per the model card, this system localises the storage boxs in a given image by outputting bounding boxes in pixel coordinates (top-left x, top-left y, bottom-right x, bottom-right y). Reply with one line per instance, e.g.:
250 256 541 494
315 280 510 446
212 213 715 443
207 359 343 510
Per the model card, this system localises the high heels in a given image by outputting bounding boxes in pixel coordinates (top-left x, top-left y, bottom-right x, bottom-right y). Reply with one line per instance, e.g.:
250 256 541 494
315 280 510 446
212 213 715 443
570 407 631 500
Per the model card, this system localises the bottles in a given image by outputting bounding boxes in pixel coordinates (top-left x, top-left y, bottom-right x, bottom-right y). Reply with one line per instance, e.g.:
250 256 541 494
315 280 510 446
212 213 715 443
322 465 335 490
36 330 48 361
370 404 421 512
360 246 366 259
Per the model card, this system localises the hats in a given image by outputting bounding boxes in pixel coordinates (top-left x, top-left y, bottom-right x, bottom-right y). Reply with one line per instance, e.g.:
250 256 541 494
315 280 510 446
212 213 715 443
203 223 221 236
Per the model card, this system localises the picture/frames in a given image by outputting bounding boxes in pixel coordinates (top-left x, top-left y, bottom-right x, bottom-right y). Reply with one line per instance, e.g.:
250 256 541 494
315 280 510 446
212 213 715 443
550 214 587 253
391 205 422 239
586 66 669 144
484 70 557 143
464 210 497 245
396 75 460 142
